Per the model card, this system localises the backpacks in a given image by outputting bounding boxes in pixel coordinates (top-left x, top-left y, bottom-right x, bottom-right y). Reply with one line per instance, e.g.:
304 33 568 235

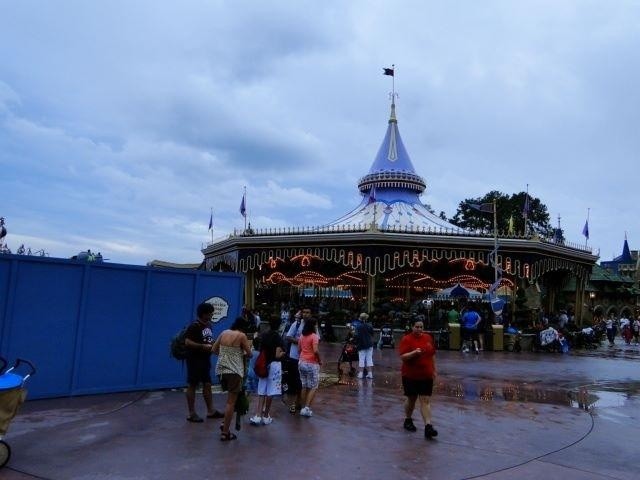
358 323 376 351
255 333 276 379
170 321 200 361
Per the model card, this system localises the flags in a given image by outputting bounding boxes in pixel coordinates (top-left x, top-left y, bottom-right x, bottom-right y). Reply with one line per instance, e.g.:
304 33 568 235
368 184 376 204
238 195 246 217
468 203 494 214
382 67 393 77
522 194 530 214
207 213 212 231
581 220 589 240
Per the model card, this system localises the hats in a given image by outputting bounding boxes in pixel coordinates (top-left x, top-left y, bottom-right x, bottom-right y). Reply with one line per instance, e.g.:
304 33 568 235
358 313 369 321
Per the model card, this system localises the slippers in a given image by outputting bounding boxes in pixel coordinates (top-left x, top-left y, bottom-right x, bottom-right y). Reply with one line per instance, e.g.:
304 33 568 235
187 414 203 422
206 410 225 419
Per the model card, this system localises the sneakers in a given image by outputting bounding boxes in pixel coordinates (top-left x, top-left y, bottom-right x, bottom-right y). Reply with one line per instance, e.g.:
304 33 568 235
424 424 438 439
300 408 310 416
249 416 261 424
366 372 373 379
476 348 479 355
462 349 469 353
403 417 416 432
357 371 363 379
262 416 273 425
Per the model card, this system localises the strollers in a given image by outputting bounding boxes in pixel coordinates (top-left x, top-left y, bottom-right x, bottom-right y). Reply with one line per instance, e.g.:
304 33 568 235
337 328 368 377
379 325 395 350
0 355 35 469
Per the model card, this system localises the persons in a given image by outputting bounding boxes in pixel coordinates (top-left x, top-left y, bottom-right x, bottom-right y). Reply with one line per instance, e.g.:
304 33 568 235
354 313 375 379
601 315 614 348
632 315 640 344
537 309 575 331
211 317 251 443
399 319 438 441
473 303 487 351
285 306 321 414
458 301 481 354
183 304 226 422
447 303 458 325
619 313 633 344
297 318 321 418
254 315 279 426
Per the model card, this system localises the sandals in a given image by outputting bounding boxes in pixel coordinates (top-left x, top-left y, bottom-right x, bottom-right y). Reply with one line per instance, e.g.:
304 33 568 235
220 431 237 441
